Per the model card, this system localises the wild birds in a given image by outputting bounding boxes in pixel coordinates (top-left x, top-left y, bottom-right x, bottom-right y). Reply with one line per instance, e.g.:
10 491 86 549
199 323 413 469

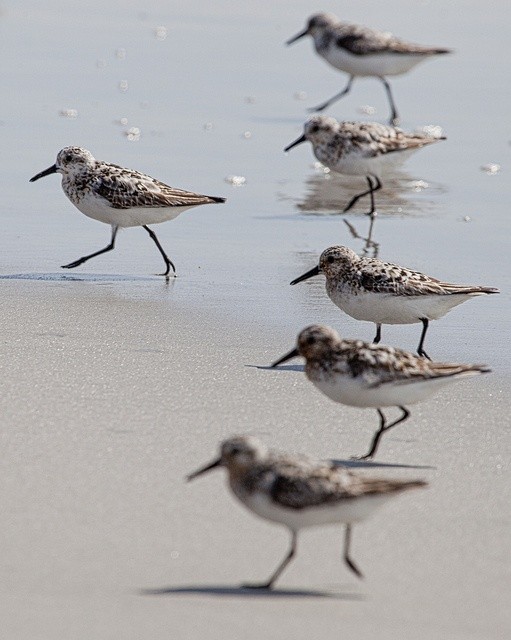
287 12 455 125
29 146 227 278
291 245 501 362
189 435 427 594
271 324 492 459
282 115 446 218
